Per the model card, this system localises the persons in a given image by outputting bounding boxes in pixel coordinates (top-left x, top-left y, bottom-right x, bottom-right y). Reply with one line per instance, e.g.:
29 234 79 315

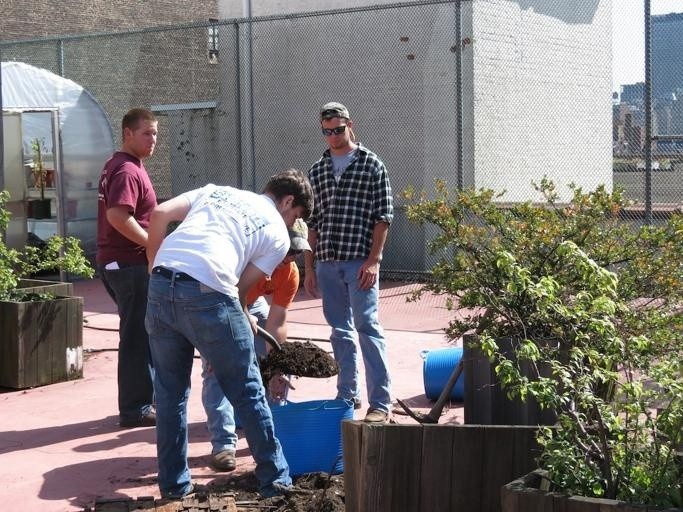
200 216 313 470
93 108 160 428
144 168 316 500
302 101 394 424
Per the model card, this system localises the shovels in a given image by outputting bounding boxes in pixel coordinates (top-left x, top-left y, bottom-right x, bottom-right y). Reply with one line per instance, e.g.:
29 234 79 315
256 325 342 378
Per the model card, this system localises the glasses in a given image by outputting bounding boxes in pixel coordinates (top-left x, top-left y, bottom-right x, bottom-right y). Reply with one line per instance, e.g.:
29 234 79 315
286 249 302 256
321 125 346 136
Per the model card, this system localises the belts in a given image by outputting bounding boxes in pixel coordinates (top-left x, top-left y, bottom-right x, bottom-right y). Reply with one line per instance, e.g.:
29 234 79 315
151 266 196 282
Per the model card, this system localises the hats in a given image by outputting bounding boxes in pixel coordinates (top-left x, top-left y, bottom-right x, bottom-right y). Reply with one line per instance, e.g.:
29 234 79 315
288 217 313 253
321 102 350 123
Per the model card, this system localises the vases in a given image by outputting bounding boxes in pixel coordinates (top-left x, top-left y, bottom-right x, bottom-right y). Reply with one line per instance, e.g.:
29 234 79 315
46 169 55 186
26 161 36 187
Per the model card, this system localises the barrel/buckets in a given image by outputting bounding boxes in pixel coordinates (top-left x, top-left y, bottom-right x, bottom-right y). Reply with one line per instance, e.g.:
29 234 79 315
267 396 356 478
422 346 466 403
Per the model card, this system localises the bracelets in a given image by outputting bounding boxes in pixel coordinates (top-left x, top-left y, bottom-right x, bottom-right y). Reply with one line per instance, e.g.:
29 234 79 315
304 269 313 271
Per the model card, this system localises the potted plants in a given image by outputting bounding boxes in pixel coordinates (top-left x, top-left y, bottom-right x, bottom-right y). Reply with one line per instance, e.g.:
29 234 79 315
28 136 51 219
398 175 683 512
0 192 94 389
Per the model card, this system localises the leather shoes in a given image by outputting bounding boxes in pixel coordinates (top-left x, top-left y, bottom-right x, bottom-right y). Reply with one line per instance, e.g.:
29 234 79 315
354 399 361 409
212 449 236 470
364 407 389 423
119 405 157 427
185 482 208 500
